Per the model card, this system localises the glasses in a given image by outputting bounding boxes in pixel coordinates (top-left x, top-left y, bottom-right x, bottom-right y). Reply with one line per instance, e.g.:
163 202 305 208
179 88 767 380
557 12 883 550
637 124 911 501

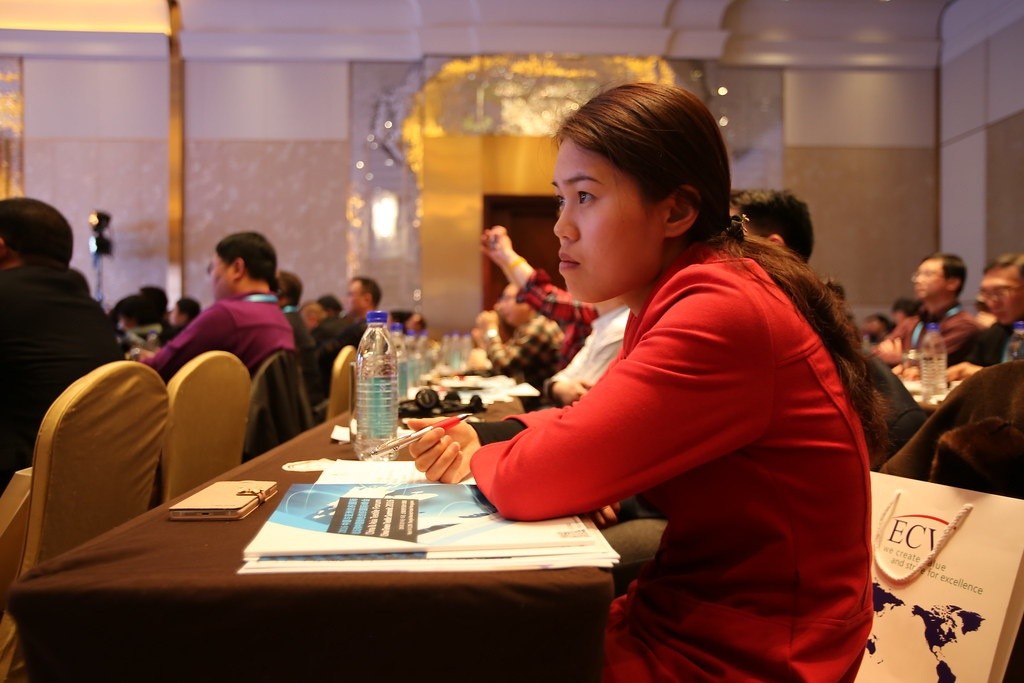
975 286 1024 302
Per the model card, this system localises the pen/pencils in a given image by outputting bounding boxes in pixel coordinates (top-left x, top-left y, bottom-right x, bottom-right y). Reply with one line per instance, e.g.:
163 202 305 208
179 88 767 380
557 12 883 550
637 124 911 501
370 414 469 456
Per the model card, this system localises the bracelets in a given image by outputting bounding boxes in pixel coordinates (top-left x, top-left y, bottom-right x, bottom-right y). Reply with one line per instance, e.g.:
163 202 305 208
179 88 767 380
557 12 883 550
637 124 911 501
504 257 527 272
483 328 501 341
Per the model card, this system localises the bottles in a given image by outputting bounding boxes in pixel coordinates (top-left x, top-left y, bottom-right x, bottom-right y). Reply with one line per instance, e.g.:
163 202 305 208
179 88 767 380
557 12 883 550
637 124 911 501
354 312 398 462
1009 322 1024 361
920 321 946 405
391 323 471 402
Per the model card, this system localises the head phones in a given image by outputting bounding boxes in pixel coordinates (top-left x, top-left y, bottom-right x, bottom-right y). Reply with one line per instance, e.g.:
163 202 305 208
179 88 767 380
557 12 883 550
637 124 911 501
399 389 483 419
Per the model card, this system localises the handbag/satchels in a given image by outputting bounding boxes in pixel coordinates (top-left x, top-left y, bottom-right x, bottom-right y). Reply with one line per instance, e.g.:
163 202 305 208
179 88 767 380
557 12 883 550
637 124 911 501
854 471 1024 683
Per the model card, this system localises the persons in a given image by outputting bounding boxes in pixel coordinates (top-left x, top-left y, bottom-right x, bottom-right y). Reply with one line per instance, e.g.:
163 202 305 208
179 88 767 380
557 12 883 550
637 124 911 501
464 190 815 580
0 195 123 495
312 276 381 362
146 230 298 384
387 309 425 337
274 270 315 347
107 284 199 349
826 250 1024 501
300 296 344 331
397 81 878 683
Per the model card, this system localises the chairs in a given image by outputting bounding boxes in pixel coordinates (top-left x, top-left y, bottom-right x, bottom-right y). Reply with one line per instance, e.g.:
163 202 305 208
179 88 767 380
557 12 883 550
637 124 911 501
244 350 316 464
0 361 170 683
324 346 359 420
154 351 251 505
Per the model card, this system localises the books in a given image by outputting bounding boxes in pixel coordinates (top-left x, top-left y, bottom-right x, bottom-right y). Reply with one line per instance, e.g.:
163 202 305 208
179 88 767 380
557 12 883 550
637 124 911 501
242 483 592 555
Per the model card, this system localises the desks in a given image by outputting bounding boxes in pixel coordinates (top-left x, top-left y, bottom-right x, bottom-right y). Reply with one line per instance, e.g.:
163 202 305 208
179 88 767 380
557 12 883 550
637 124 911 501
4 368 613 683
